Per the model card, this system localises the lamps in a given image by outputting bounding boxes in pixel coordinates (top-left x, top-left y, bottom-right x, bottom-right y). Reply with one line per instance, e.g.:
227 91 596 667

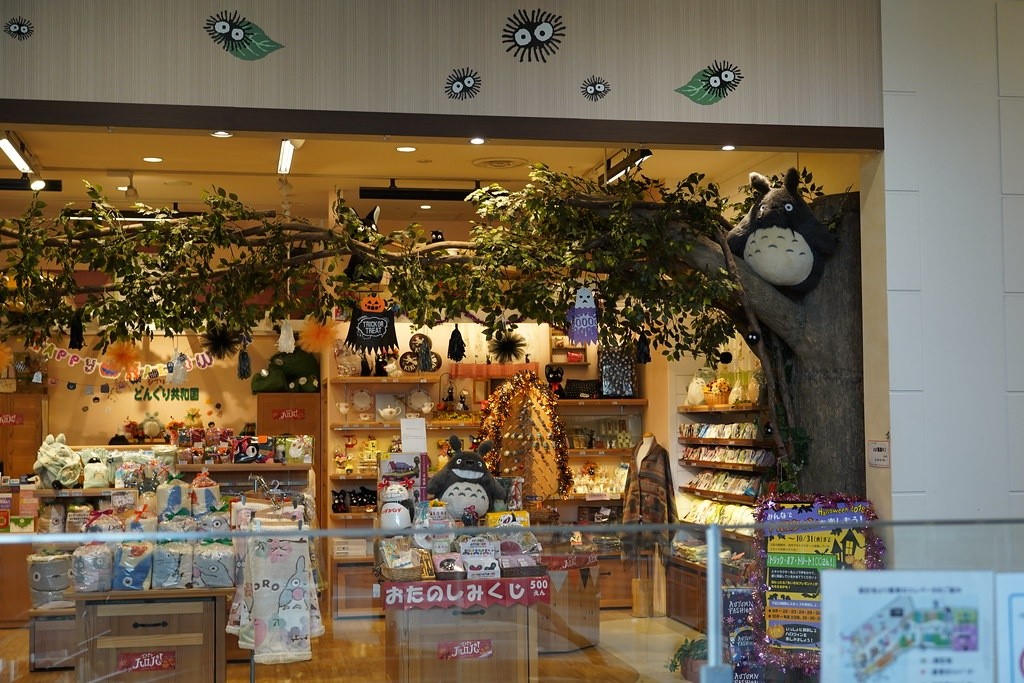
1 131 740 227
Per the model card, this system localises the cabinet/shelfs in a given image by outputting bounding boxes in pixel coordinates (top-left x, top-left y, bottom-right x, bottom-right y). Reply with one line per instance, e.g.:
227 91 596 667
0 542 33 630
672 395 773 570
661 553 708 635
255 389 321 442
62 587 233 683
536 559 599 654
546 315 593 372
381 575 547 683
28 601 251 673
323 549 385 618
325 344 651 549
0 391 49 483
596 550 633 609
29 442 317 552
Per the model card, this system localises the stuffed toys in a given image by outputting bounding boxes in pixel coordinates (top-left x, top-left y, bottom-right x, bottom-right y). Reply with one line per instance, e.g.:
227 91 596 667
32 431 82 490
684 356 718 407
136 411 165 436
726 167 838 295
425 435 507 520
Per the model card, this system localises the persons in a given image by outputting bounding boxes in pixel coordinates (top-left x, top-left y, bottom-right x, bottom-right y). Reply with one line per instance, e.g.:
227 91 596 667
630 432 666 534
